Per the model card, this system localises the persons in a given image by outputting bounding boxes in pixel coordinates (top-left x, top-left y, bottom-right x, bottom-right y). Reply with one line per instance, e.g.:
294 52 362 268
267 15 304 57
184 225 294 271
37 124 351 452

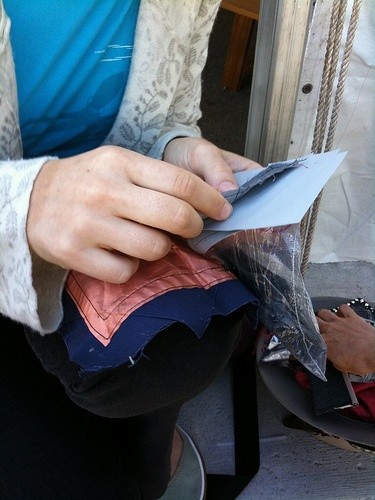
0 0 271 500
315 304 375 378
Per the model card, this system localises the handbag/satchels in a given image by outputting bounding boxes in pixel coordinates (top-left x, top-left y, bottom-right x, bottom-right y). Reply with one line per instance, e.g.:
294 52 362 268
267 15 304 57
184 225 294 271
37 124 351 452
295 299 375 422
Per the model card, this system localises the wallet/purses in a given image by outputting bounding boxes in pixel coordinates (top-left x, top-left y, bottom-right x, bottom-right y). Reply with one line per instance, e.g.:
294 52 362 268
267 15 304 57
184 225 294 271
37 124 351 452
306 357 360 416
